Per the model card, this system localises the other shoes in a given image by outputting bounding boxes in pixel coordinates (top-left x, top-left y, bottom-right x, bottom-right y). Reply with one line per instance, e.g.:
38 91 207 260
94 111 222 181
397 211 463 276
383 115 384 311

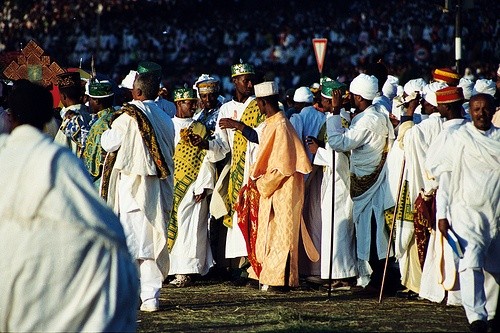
323 280 352 291
140 298 159 311
306 275 323 283
261 285 273 291
169 276 188 287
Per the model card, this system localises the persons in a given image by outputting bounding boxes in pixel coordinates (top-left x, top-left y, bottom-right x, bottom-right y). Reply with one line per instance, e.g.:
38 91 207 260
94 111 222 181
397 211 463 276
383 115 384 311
0 0 500 332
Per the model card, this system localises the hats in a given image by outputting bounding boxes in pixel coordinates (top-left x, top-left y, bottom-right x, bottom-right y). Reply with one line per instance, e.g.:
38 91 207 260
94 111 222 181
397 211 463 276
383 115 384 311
88 79 115 98
433 68 461 85
349 73 378 101
174 89 199 101
55 71 83 88
193 74 220 94
422 80 449 105
436 87 462 103
404 78 426 95
321 77 348 98
136 61 161 76
292 87 313 102
254 81 281 97
231 58 256 77
471 79 496 96
